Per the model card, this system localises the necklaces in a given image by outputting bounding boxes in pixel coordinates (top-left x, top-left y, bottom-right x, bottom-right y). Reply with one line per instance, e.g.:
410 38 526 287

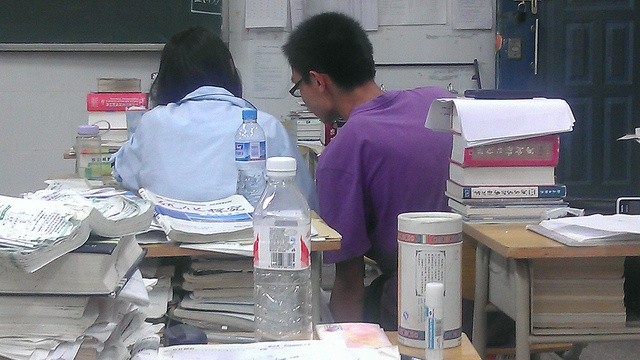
107 26 319 216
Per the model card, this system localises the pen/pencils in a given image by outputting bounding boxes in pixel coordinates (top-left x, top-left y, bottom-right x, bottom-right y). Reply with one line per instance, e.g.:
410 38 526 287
205 333 257 344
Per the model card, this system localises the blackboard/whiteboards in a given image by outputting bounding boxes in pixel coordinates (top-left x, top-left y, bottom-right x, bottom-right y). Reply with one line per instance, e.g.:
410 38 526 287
0 0 225 46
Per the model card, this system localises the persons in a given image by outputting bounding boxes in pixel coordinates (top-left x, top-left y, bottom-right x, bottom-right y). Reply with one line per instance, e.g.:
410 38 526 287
281 10 467 331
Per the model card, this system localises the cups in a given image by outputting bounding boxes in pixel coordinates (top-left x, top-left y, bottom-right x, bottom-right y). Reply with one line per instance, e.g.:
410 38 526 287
74 119 105 181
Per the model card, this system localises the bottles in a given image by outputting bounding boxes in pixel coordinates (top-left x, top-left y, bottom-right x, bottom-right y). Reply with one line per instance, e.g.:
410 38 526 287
252 157 312 341
236 108 265 212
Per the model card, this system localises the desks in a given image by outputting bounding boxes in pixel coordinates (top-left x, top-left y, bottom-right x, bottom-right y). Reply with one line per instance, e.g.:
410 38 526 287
16 191 341 335
463 210 640 358
63 77 282 165
387 330 481 360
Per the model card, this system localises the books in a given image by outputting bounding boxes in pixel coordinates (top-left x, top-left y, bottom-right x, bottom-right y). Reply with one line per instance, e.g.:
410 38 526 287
86 77 148 148
529 257 627 333
444 96 570 225
524 212 640 248
287 110 325 145
0 179 345 360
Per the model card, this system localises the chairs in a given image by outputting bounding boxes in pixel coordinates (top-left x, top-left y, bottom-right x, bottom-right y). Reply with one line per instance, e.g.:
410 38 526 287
465 220 588 360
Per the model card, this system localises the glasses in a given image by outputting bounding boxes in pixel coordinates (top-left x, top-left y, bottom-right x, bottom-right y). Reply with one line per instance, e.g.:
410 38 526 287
289 73 309 97
